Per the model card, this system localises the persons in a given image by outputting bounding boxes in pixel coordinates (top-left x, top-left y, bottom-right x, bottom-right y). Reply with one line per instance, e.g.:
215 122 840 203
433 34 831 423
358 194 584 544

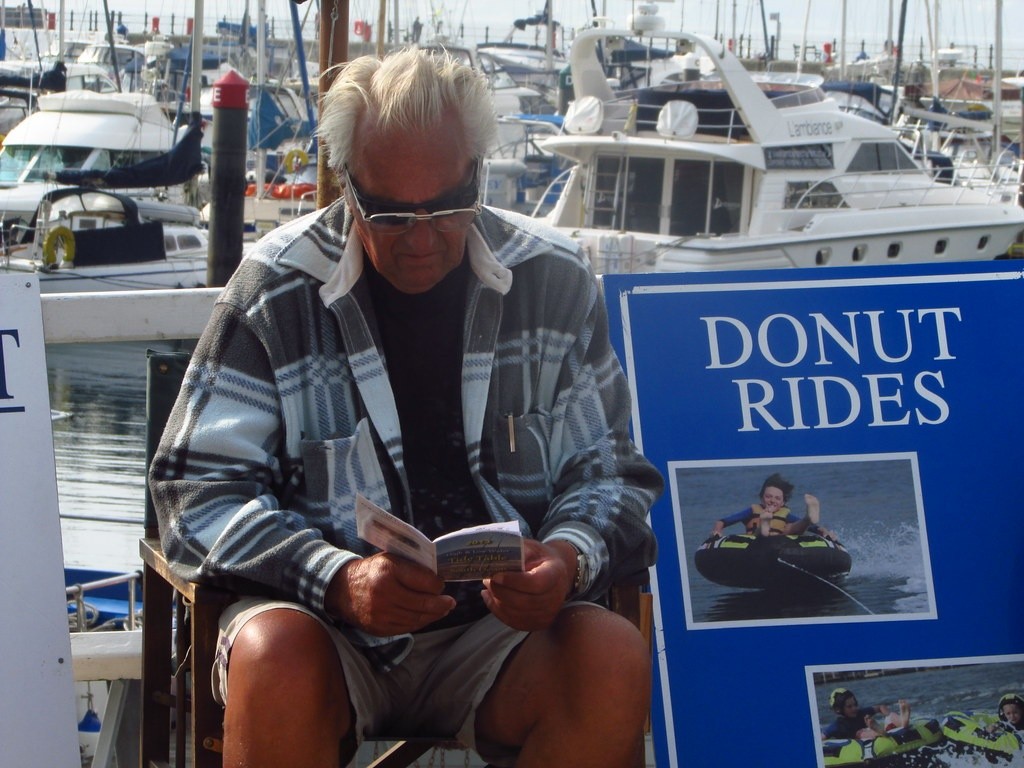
987 692 1024 733
709 471 839 542
148 44 666 768
820 687 911 740
412 16 424 42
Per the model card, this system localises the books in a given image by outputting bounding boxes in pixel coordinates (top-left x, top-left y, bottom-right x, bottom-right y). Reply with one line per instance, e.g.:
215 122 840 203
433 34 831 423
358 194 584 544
353 490 524 583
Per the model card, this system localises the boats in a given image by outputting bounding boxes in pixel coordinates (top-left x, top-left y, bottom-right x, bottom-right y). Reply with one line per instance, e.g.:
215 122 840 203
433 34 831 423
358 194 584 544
513 0 1023 275
939 711 1024 766
693 533 853 590
822 716 943 768
0 87 206 234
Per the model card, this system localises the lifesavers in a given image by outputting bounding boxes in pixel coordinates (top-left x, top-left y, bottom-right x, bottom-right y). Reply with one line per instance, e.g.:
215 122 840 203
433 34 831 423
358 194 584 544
41 225 75 271
287 149 308 175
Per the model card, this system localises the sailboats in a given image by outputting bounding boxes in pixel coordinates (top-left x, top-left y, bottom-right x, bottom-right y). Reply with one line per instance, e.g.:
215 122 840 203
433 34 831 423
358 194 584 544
0 0 1024 208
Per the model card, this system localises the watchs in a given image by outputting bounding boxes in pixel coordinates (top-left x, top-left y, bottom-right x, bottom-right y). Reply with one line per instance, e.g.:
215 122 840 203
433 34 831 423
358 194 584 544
563 538 590 604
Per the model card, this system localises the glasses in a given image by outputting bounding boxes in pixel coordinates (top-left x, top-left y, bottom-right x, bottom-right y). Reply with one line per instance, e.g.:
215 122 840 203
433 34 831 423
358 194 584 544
344 162 489 235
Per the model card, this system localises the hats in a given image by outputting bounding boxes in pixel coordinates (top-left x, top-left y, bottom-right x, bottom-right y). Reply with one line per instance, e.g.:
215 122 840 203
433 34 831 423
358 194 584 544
53 61 67 72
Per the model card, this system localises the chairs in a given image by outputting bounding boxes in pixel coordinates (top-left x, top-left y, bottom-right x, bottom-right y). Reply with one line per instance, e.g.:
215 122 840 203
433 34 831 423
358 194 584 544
135 347 433 768
